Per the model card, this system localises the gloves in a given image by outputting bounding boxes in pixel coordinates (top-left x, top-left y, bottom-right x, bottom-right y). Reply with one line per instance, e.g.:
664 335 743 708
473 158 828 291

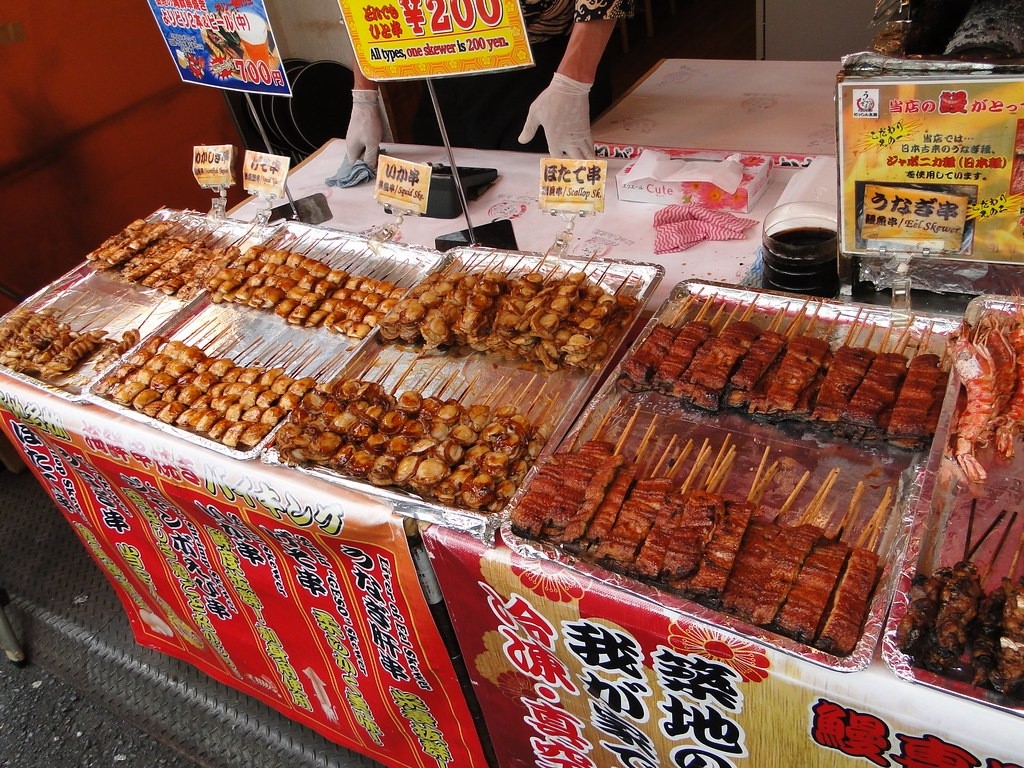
346 88 381 167
518 72 596 159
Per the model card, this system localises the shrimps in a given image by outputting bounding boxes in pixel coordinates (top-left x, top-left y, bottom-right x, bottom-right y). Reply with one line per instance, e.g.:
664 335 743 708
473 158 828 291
940 288 1024 484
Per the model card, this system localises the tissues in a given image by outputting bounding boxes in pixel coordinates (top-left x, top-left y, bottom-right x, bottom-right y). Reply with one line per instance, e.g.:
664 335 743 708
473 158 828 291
619 146 776 215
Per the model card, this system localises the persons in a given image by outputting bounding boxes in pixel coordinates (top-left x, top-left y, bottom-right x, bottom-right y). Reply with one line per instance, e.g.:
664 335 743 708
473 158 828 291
346 0 632 172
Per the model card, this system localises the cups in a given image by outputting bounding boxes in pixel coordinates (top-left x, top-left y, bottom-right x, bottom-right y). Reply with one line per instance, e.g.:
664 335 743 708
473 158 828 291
763 200 845 300
